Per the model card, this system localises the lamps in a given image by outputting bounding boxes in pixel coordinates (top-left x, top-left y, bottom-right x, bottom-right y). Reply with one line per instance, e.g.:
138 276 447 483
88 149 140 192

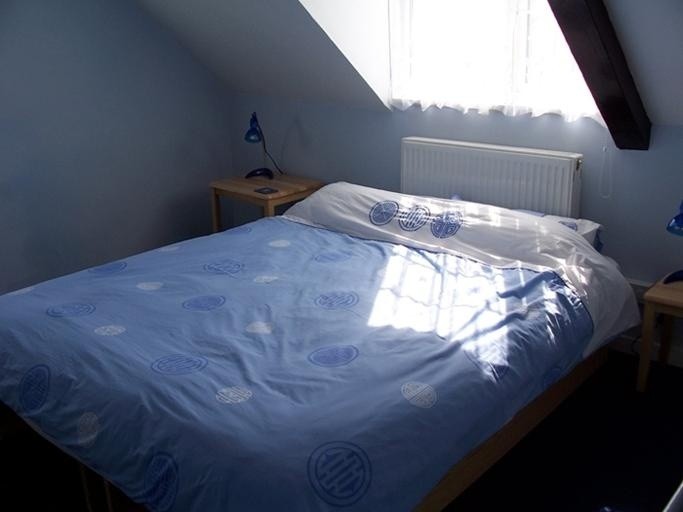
663 200 683 284
243 113 284 179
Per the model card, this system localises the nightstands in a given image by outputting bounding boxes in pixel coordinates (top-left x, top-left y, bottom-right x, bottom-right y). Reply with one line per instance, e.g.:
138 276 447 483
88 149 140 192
211 174 323 233
637 271 683 392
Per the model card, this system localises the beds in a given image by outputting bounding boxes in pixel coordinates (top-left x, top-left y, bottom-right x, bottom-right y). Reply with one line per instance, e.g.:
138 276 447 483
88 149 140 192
0 134 605 512
